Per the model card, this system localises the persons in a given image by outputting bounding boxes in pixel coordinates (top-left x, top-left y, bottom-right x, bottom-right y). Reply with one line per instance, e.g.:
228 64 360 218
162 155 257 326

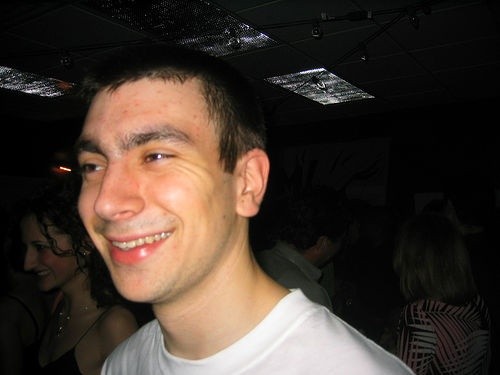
0 179 139 375
0 240 50 375
75 49 416 375
254 183 349 312
392 213 492 375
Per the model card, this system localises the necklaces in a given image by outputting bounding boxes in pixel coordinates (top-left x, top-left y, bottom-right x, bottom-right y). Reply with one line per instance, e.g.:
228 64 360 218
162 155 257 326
56 300 98 337
